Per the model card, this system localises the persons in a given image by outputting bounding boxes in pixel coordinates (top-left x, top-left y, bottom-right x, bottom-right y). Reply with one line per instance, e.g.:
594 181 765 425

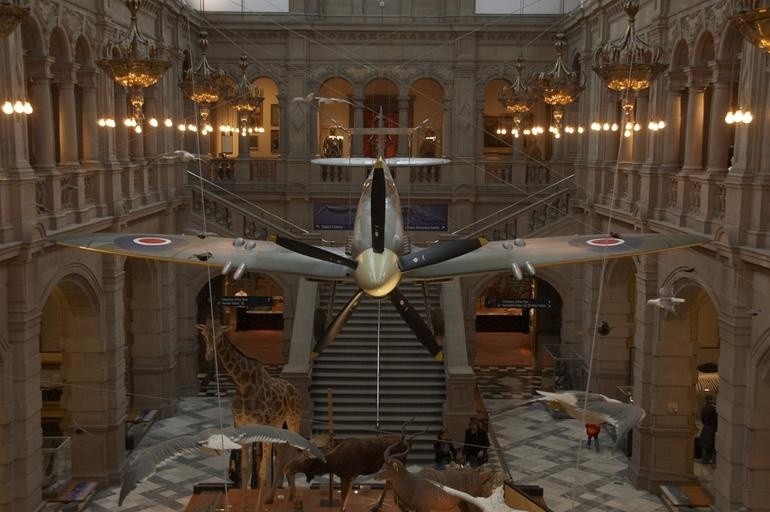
697 395 718 465
321 125 346 183
462 417 490 465
419 122 439 177
585 420 602 451
435 429 457 465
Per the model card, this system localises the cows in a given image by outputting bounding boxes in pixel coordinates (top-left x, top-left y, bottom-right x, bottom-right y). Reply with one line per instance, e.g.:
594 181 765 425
304 431 407 512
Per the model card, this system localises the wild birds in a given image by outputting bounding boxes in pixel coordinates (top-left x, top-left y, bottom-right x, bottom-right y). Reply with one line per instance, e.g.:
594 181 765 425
117 423 329 508
534 388 647 455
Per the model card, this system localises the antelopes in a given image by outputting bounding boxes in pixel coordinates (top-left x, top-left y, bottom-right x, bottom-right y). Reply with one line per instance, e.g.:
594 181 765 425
373 413 484 512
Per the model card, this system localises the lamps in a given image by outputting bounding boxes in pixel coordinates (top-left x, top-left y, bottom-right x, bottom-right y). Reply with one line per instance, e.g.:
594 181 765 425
496 1 670 139
93 0 265 136
730 1 770 54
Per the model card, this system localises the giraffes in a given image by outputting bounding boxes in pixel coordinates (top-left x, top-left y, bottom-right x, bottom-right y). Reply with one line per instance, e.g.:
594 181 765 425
195 317 304 511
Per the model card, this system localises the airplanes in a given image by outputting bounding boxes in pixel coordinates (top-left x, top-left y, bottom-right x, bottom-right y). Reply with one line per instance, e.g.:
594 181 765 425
55 156 715 363
